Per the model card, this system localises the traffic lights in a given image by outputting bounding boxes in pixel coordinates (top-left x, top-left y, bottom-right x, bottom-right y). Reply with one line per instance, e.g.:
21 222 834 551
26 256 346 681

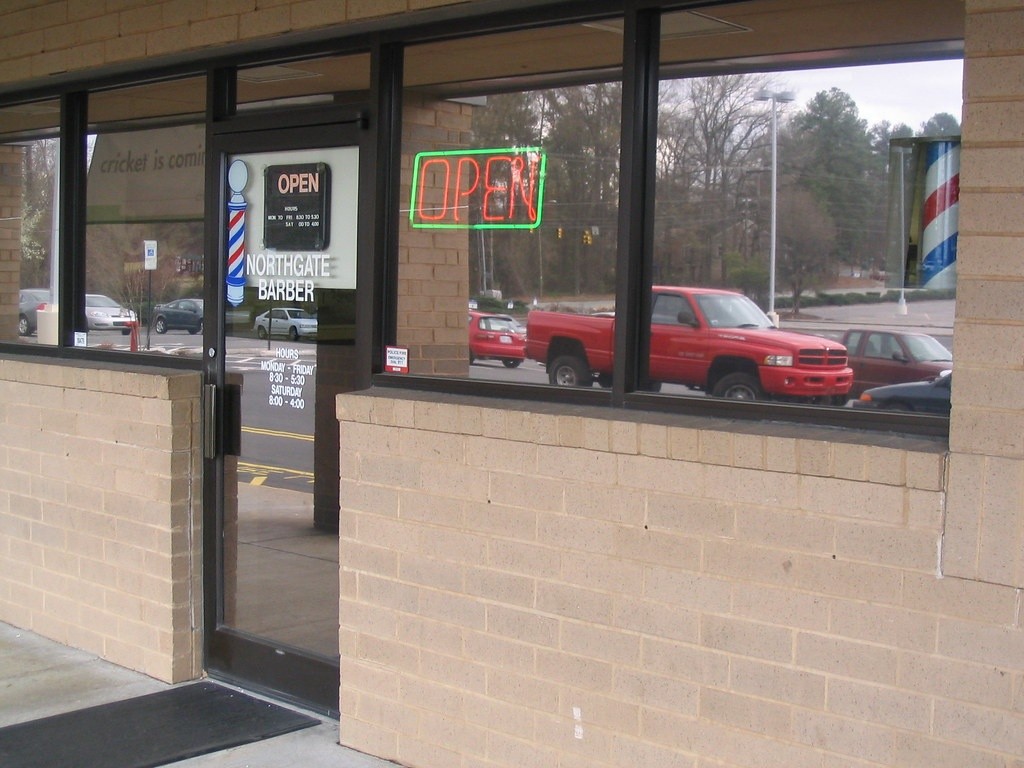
581 229 592 245
557 228 563 239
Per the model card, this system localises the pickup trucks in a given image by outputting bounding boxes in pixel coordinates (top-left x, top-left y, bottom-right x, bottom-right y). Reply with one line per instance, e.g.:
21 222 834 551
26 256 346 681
523 285 852 401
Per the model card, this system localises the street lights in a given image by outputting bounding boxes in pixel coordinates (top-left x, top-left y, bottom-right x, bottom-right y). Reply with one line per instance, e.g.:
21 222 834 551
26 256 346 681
751 89 799 328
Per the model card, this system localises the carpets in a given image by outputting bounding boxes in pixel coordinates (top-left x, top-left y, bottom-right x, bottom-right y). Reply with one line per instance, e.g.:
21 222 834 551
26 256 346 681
0 680 320 768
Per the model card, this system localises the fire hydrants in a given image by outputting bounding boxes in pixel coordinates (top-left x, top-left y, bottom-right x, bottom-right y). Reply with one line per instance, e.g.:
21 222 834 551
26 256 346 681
123 319 139 352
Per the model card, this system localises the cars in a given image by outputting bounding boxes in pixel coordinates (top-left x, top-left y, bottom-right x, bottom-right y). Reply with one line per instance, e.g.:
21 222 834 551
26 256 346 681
152 297 204 335
84 294 138 335
852 370 952 417
818 327 950 400
868 270 887 282
18 289 50 336
254 308 317 341
467 308 527 369
